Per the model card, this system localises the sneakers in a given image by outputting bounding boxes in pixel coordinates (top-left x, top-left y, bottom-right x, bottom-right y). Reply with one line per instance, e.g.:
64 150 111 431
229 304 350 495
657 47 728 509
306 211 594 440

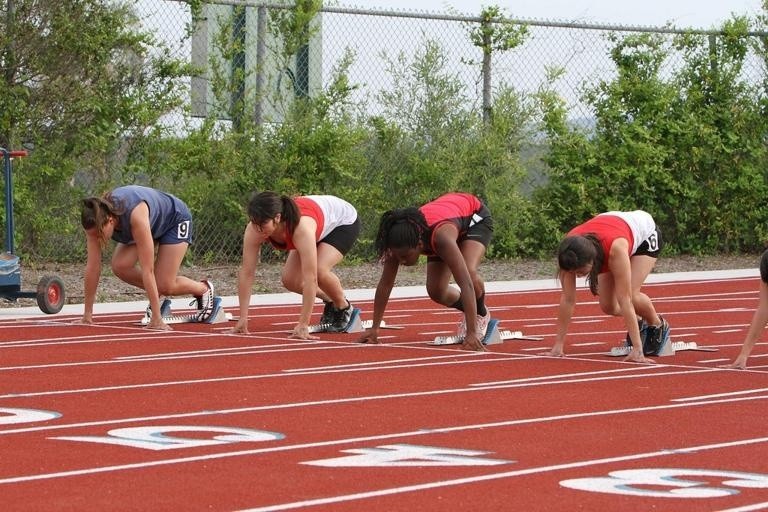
319 301 333 324
640 315 670 356
327 299 354 333
189 279 214 322
145 295 165 324
462 304 491 341
626 318 643 346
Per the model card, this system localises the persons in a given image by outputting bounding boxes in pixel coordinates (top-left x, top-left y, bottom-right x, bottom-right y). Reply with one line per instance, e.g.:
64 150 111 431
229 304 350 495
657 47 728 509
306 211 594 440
356 192 494 352
537 210 671 365
71 185 214 331
715 246 767 371
220 191 361 339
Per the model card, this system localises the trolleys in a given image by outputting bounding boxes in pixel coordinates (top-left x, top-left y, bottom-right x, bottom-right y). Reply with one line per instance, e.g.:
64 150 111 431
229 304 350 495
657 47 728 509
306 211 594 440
0 144 65 314
